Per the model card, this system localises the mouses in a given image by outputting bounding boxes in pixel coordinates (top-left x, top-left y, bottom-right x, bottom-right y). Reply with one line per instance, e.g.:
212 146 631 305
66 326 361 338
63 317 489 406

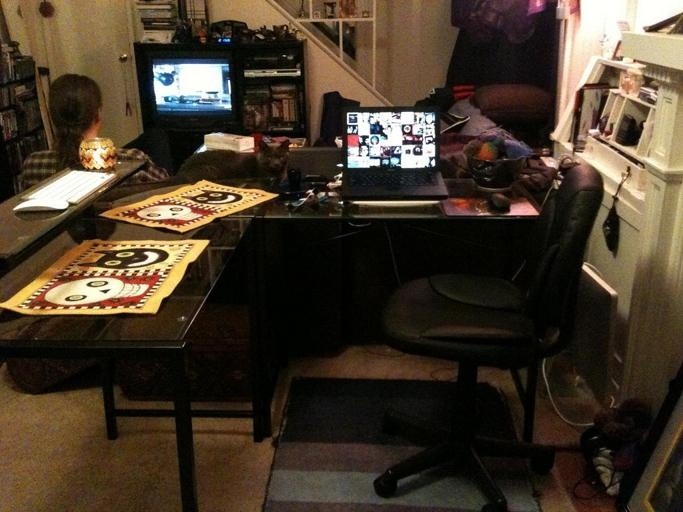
489 192 511 212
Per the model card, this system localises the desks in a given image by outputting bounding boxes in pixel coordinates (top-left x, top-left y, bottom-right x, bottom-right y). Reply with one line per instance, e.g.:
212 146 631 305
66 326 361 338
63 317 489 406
0 177 544 512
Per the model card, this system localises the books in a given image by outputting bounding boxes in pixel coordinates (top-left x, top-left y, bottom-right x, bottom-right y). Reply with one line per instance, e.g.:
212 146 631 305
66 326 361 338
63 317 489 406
0 41 46 196
244 83 302 127
135 0 178 44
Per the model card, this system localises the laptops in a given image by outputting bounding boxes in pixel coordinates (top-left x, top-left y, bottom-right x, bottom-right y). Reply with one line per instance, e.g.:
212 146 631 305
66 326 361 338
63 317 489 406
340 106 450 200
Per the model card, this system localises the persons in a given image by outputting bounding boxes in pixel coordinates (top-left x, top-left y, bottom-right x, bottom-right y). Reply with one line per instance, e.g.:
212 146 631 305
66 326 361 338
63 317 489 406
22 73 169 193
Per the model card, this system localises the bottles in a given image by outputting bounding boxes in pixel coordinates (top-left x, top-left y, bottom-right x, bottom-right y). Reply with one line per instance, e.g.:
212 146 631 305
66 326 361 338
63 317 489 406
618 67 641 99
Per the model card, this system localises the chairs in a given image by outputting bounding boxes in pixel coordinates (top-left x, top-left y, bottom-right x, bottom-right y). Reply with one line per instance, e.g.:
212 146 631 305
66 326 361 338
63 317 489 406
373 162 605 512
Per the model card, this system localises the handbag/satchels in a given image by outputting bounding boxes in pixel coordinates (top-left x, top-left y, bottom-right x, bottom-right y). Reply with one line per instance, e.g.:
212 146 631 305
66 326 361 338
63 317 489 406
581 427 638 497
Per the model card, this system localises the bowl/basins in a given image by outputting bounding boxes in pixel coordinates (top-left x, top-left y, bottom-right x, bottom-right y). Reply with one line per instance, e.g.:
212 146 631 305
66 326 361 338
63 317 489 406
463 140 532 193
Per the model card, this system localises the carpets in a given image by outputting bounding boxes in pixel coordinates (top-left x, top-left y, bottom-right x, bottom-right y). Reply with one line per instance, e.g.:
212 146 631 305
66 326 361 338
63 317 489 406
4 304 288 443
261 375 544 512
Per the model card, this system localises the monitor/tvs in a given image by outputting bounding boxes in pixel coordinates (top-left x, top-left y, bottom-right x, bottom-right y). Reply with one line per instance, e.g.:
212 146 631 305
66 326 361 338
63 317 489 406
144 50 235 121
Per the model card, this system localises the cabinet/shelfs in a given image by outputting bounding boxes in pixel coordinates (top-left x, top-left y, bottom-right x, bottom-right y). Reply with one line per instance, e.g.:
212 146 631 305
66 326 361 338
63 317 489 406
577 54 683 452
134 36 312 147
0 73 50 205
